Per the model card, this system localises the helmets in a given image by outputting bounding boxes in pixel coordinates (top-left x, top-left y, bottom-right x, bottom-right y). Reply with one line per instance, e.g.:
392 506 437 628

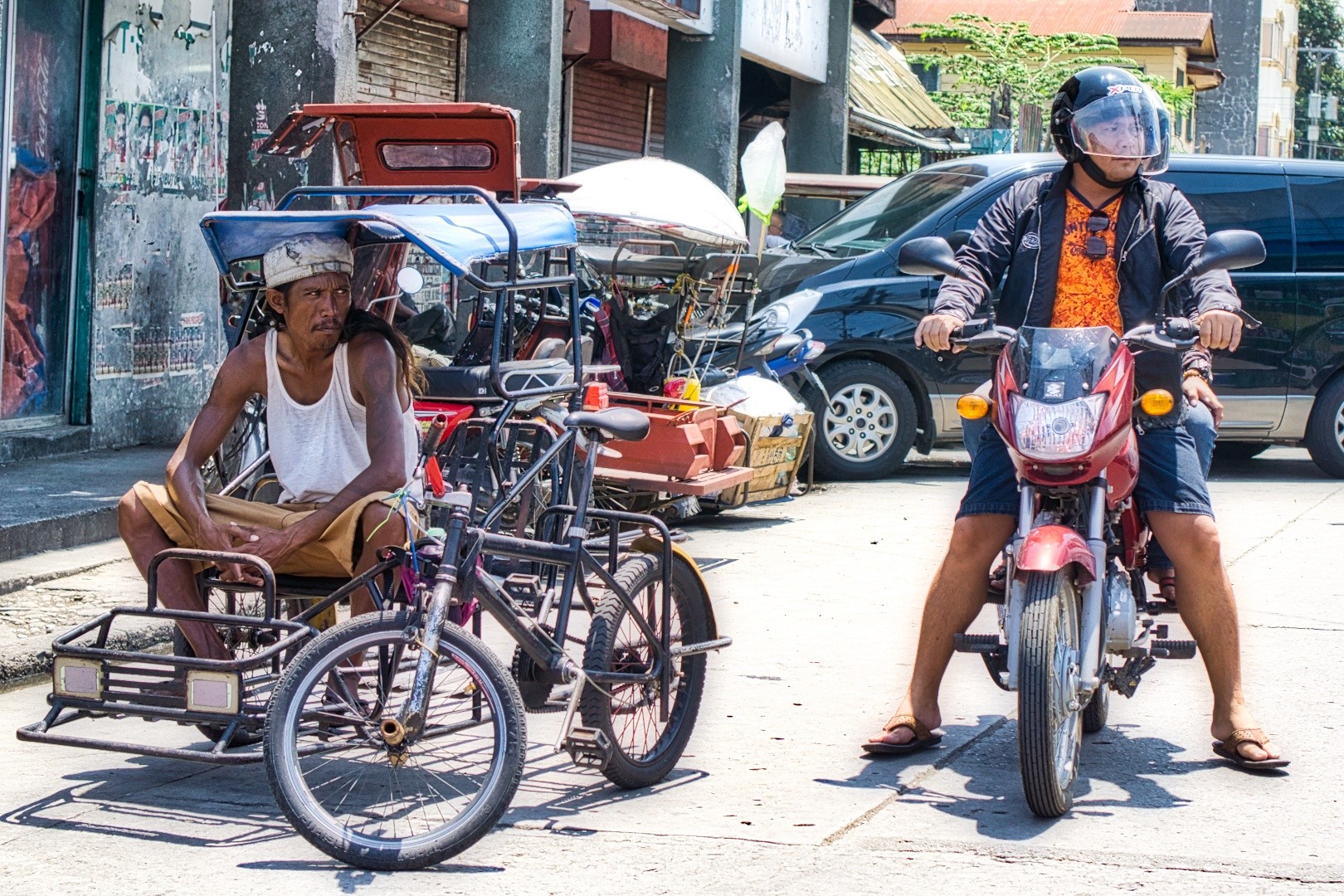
1051 66 1170 163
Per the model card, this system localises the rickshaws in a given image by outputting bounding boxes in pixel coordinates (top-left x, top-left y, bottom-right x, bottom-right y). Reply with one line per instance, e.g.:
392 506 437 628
15 406 733 871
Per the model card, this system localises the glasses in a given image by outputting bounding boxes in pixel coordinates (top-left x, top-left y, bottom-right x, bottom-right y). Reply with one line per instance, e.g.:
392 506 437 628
1083 209 1111 258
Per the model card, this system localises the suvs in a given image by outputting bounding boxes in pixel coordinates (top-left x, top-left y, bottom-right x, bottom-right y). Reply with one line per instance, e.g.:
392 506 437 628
668 151 1344 479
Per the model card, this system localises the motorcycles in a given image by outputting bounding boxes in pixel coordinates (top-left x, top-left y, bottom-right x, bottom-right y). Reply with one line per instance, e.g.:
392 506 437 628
573 288 839 417
895 229 1269 820
196 184 754 524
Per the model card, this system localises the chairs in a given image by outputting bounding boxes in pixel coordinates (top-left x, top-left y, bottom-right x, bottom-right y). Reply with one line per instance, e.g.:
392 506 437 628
193 475 352 610
447 261 506 366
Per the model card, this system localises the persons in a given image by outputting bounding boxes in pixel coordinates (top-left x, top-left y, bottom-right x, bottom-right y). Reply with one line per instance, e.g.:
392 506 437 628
116 234 423 728
860 65 1288 772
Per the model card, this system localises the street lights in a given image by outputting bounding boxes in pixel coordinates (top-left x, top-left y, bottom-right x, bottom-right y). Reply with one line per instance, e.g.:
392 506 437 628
1307 93 1338 159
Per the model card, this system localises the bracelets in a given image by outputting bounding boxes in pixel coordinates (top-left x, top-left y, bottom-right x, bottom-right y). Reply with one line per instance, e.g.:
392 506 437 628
1179 369 1209 385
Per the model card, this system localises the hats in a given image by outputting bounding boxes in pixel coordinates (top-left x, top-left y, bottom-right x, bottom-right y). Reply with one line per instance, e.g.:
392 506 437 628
262 232 354 290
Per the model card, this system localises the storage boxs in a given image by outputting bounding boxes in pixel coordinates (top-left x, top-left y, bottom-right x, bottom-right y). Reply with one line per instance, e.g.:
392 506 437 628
699 385 815 505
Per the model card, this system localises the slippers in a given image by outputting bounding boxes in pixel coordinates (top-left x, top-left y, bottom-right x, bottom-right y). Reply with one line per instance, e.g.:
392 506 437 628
1212 727 1291 768
862 715 942 755
987 564 1007 596
1153 578 1179 610
